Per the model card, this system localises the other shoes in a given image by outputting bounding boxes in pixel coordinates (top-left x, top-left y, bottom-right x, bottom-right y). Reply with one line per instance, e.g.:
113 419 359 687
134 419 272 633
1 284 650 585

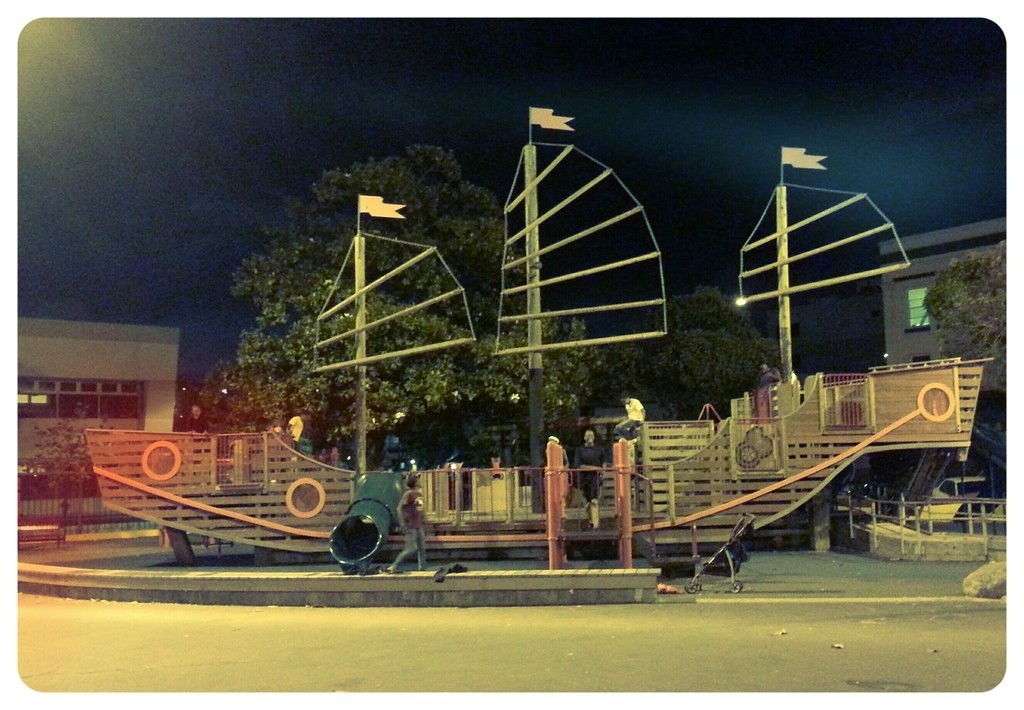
384 568 404 574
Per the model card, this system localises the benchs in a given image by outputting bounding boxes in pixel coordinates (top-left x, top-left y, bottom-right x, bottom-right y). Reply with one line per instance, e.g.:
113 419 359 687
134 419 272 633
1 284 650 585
18 514 66 550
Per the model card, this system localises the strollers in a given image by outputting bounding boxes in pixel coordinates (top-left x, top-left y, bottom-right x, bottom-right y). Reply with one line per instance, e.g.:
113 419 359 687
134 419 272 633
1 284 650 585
684 511 757 595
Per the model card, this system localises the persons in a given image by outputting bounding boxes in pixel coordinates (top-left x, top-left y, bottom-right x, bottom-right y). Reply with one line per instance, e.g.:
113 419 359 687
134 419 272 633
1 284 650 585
755 361 797 389
615 392 646 445
386 471 426 573
574 428 606 532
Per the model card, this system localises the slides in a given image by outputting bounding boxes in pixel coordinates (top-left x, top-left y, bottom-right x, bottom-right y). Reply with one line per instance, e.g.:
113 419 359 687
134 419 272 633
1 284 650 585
330 474 403 574
